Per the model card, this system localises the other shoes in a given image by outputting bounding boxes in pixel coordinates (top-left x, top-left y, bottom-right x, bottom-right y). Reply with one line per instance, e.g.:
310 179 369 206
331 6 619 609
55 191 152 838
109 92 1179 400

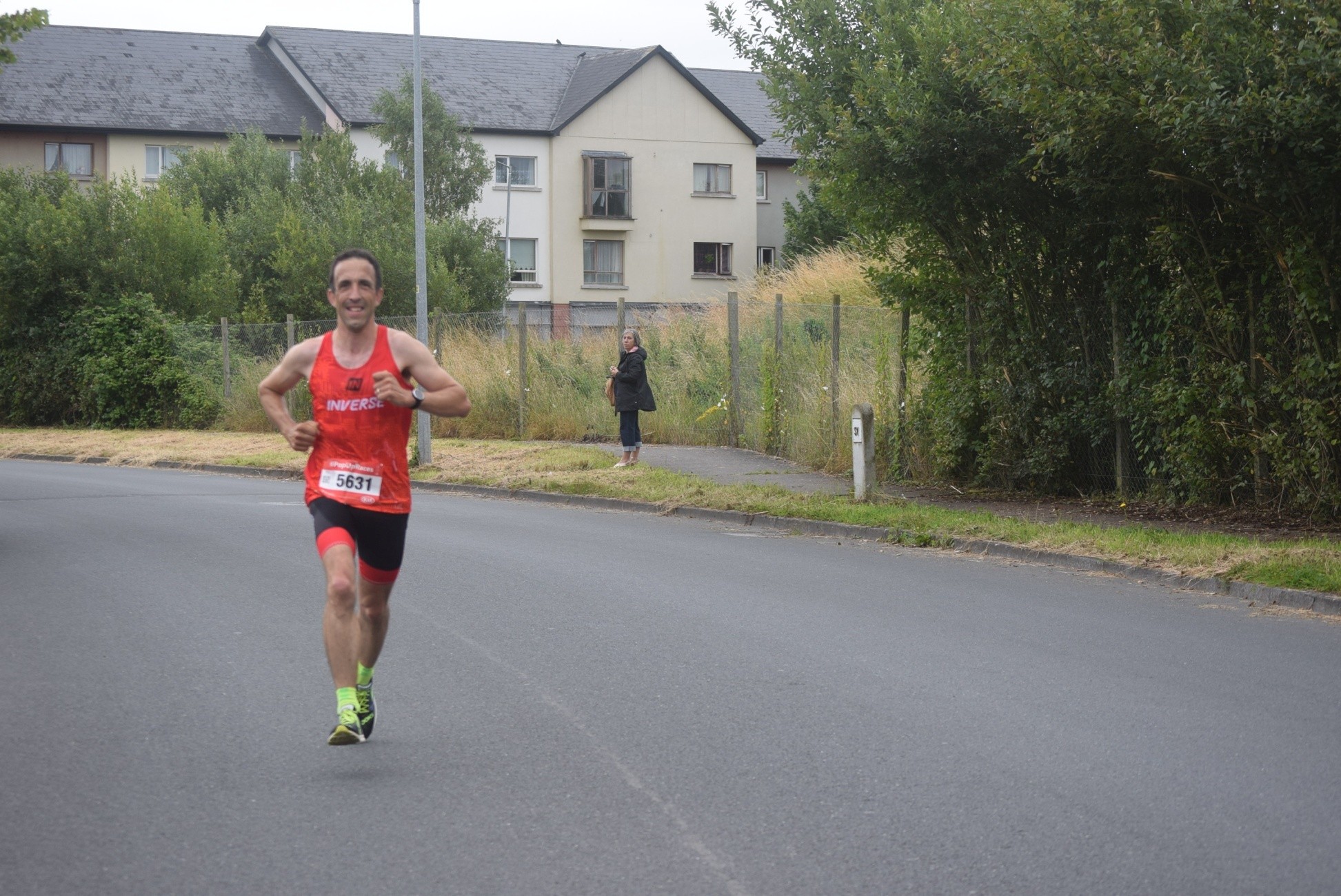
613 462 630 469
629 457 639 466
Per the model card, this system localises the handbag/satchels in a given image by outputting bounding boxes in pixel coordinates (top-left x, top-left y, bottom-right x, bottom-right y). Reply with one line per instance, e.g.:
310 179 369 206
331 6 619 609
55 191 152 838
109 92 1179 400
605 377 615 406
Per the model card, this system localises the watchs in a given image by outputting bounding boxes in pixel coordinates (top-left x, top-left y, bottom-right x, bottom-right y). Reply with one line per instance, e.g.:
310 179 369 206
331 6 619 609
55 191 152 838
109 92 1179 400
409 388 425 409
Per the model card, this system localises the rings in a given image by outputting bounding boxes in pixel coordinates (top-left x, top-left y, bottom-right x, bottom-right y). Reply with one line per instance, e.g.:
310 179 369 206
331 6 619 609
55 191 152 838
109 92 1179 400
377 388 380 394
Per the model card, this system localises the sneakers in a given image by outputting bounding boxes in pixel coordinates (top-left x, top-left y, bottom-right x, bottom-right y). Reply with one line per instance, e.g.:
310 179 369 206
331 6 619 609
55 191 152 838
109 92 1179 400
355 678 375 738
328 705 360 745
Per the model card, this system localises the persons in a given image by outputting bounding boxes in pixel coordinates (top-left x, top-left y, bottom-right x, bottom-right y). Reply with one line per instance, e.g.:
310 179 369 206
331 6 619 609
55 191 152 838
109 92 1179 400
611 328 657 468
258 248 473 745
